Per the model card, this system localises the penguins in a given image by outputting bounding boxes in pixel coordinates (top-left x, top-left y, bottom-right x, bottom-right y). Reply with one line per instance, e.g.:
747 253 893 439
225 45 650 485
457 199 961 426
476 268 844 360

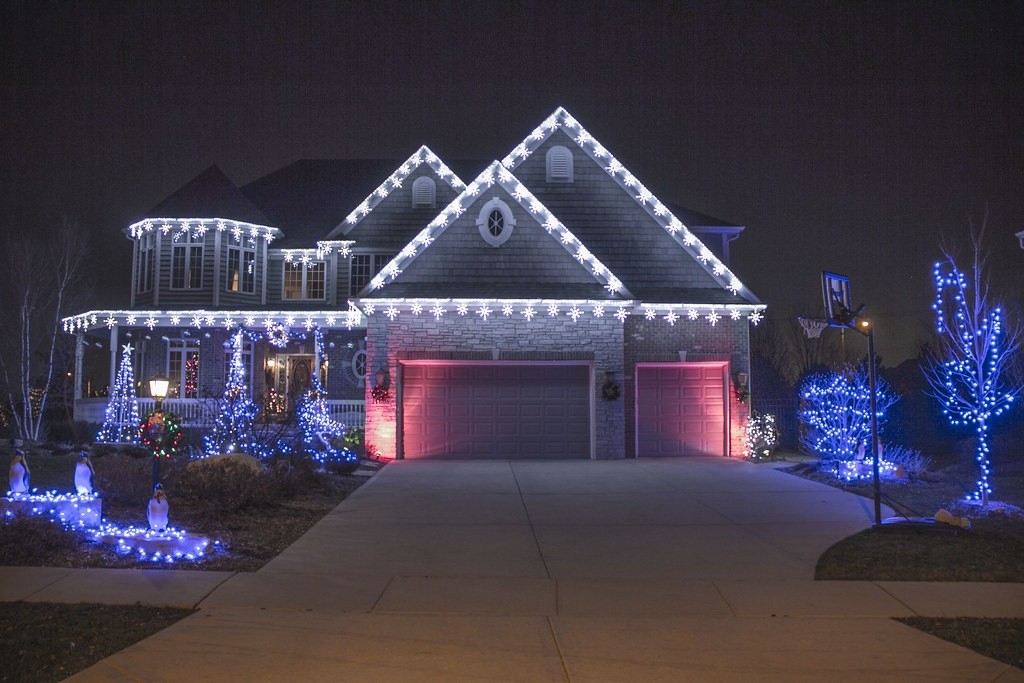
854 437 883 460
73 452 95 495
146 482 169 533
9 449 30 495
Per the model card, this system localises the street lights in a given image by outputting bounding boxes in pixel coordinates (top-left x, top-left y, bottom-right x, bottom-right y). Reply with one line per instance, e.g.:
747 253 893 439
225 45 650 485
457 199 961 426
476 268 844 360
145 372 171 527
862 319 881 526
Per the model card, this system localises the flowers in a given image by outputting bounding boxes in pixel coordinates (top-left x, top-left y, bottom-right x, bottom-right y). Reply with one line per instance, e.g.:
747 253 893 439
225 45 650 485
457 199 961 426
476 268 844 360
736 388 752 404
371 388 390 404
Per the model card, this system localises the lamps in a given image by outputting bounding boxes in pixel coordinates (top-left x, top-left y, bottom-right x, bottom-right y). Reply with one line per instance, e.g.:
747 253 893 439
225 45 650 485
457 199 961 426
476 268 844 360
375 368 386 388
737 367 749 386
605 372 615 385
148 371 169 398
